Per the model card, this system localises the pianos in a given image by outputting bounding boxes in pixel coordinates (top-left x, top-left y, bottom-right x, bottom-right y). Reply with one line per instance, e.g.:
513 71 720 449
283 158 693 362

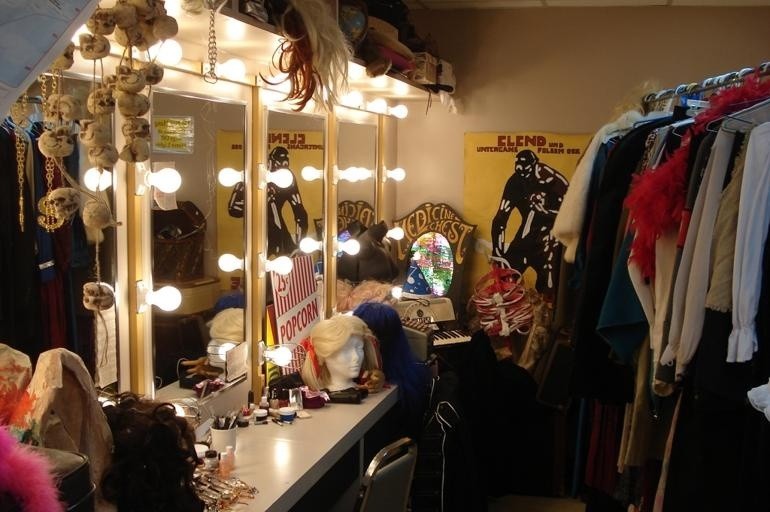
398 298 472 351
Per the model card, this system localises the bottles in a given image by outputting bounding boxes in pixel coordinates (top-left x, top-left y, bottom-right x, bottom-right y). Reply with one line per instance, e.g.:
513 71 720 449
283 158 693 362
206 447 235 475
237 388 303 427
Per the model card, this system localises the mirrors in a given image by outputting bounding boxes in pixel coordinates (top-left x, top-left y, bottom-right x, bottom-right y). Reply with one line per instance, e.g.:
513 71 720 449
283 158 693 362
333 118 380 313
145 85 253 416
2 71 119 412
257 107 326 374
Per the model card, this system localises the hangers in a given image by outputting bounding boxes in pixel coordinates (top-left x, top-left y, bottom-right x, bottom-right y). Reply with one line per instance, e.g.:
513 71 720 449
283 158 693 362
637 60 770 141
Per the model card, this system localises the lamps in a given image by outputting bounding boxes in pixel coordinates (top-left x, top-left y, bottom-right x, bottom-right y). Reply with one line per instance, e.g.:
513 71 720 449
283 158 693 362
381 164 405 183
218 251 245 272
385 226 405 241
331 236 360 257
139 37 411 121
218 166 245 188
257 252 293 279
135 278 182 322
302 165 324 182
130 158 180 198
358 167 375 181
204 336 241 369
83 166 113 193
334 164 360 184
257 162 294 193
258 338 307 371
299 236 324 254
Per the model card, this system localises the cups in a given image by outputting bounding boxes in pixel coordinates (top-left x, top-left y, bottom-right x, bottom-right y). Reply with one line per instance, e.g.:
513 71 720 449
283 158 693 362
210 425 236 453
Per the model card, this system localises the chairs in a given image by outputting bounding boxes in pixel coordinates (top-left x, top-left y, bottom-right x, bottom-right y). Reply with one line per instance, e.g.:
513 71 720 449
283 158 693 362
356 437 418 512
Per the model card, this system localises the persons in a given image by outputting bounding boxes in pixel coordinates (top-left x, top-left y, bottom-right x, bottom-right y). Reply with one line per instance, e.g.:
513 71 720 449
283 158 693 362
300 315 383 394
